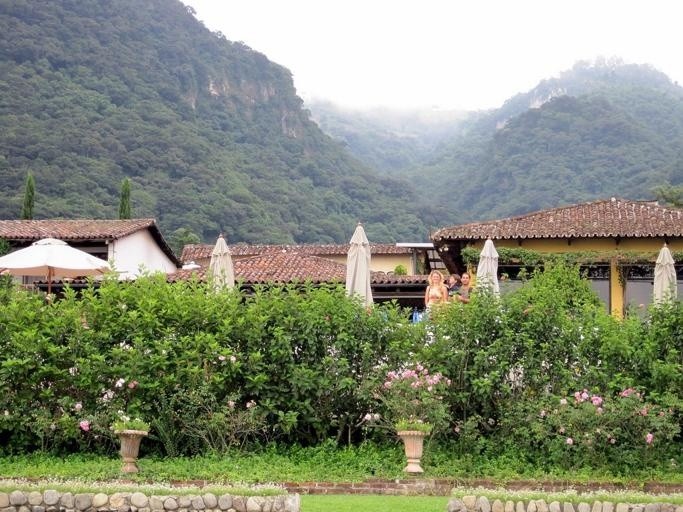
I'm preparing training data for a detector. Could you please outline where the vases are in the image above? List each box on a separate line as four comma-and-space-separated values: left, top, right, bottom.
397, 431, 429, 473
114, 430, 147, 473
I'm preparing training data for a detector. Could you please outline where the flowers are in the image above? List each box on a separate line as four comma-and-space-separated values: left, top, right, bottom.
108, 416, 151, 432
365, 362, 451, 434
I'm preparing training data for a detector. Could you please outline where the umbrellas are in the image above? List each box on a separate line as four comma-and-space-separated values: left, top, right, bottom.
652, 246, 677, 311
205, 231, 235, 302
345, 218, 375, 310
0, 237, 111, 305
472, 239, 500, 301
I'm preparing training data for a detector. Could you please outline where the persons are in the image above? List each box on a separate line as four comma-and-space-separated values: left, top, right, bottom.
423, 269, 448, 321
454, 272, 474, 305
445, 273, 461, 296
